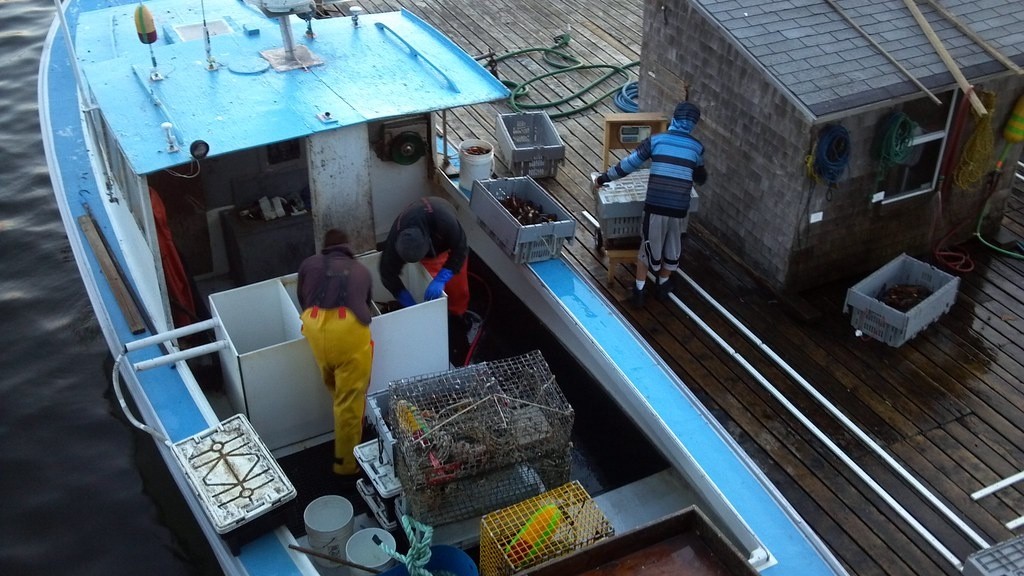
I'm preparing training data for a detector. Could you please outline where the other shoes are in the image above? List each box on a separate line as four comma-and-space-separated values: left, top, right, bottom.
352, 466, 367, 475
633, 280, 648, 296
655, 278, 671, 290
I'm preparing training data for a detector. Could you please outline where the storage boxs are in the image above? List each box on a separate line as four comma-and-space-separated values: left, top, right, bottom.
172, 413, 298, 557
843, 252, 961, 347
495, 109, 566, 179
590, 167, 698, 238
352, 440, 548, 550
469, 175, 576, 265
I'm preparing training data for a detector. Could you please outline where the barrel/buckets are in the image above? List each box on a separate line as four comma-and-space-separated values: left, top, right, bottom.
459, 139, 495, 192
303, 494, 397, 576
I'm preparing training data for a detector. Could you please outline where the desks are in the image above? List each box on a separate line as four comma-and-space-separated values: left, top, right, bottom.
220, 205, 316, 286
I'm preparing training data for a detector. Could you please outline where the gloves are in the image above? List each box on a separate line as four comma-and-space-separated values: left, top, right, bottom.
400, 292, 416, 307
424, 268, 454, 302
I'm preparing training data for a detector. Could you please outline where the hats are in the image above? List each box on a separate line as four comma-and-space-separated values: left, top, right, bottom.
396, 228, 424, 263
324, 229, 348, 247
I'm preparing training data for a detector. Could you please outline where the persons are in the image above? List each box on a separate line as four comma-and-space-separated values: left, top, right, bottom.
296, 229, 374, 494
594, 102, 707, 304
379, 197, 469, 353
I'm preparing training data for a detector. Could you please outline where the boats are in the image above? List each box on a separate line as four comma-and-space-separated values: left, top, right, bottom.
34, 1, 854, 576
